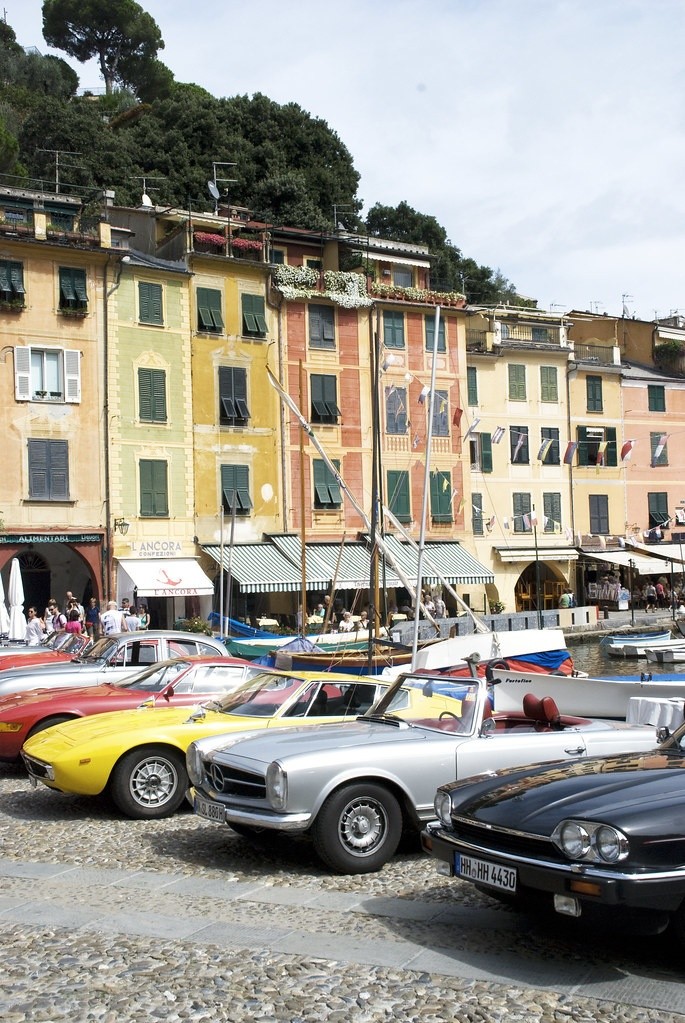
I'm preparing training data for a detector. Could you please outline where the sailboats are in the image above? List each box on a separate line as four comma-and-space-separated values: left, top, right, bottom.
206, 305, 591, 710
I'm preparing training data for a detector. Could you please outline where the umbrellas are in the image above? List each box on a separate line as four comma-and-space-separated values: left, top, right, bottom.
0, 558, 27, 641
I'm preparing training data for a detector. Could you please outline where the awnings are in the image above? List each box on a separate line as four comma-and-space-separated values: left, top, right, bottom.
583, 545, 685, 575
116, 559, 215, 596
501, 555, 578, 562
202, 536, 495, 593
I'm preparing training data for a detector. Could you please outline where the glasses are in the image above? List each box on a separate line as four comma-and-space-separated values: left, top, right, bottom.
140, 607, 144, 609
29, 611, 33, 614
49, 609, 53, 612
122, 602, 126, 604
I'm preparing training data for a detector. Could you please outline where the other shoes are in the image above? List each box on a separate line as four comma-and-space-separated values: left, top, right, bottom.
652, 611, 655, 613
645, 609, 648, 613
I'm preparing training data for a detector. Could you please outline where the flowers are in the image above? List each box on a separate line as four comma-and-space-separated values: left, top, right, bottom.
274, 264, 374, 309
369, 280, 467, 302
193, 231, 263, 253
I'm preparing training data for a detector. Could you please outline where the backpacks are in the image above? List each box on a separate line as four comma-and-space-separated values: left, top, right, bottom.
656, 588, 659, 592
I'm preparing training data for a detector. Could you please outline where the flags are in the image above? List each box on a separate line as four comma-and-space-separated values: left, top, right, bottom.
379, 345, 447, 447
433, 409, 685, 548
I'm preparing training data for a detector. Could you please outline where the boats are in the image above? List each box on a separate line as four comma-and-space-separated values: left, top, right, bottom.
599, 629, 671, 658
675, 611, 685, 637
490, 663, 685, 722
606, 638, 685, 665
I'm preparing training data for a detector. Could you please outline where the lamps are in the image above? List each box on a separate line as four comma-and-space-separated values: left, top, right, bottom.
625, 523, 640, 536
114, 518, 129, 535
473, 518, 492, 533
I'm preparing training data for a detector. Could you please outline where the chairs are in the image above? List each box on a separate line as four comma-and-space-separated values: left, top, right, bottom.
461, 691, 492, 720
509, 694, 561, 733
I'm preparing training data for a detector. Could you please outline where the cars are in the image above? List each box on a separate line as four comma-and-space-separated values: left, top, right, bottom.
0, 634, 188, 671
0, 653, 344, 765
185, 672, 675, 874
19, 671, 499, 821
421, 718, 685, 972
1, 629, 245, 698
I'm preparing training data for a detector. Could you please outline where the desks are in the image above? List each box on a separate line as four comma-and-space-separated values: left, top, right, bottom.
585, 596, 643, 610
515, 581, 567, 611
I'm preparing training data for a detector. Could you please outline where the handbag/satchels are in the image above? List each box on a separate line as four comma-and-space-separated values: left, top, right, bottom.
569, 601, 574, 608
86, 621, 93, 628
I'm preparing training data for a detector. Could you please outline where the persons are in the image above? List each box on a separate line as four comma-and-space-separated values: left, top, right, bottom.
26, 591, 150, 646
339, 601, 370, 632
645, 576, 685, 612
419, 594, 447, 620
389, 603, 416, 619
558, 587, 575, 608
297, 595, 337, 627
